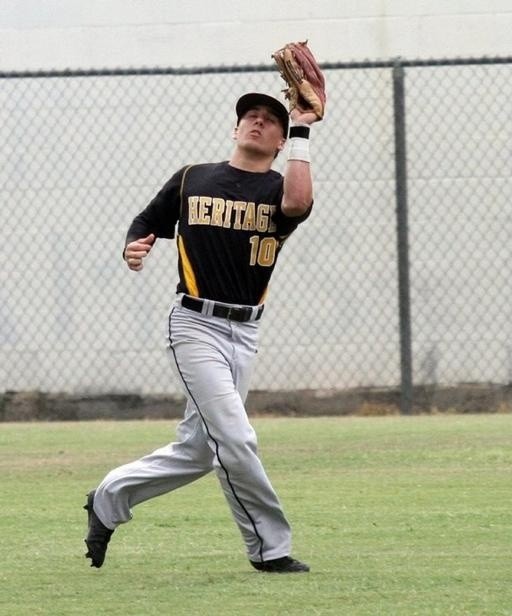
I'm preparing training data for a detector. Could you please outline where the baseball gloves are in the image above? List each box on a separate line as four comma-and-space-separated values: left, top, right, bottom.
273, 41, 326, 123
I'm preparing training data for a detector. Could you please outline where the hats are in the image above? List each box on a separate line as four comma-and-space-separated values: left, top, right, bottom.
235, 93, 288, 139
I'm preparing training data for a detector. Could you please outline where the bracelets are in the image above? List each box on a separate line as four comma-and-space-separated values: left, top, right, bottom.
287, 124, 311, 163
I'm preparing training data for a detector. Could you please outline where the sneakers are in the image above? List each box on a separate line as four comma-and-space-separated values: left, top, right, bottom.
84, 490, 114, 568
248, 555, 310, 572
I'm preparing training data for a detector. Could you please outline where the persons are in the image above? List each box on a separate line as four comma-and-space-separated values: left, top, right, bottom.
83, 92, 314, 573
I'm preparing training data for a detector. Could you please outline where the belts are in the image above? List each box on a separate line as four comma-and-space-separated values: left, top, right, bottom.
181, 294, 264, 323
